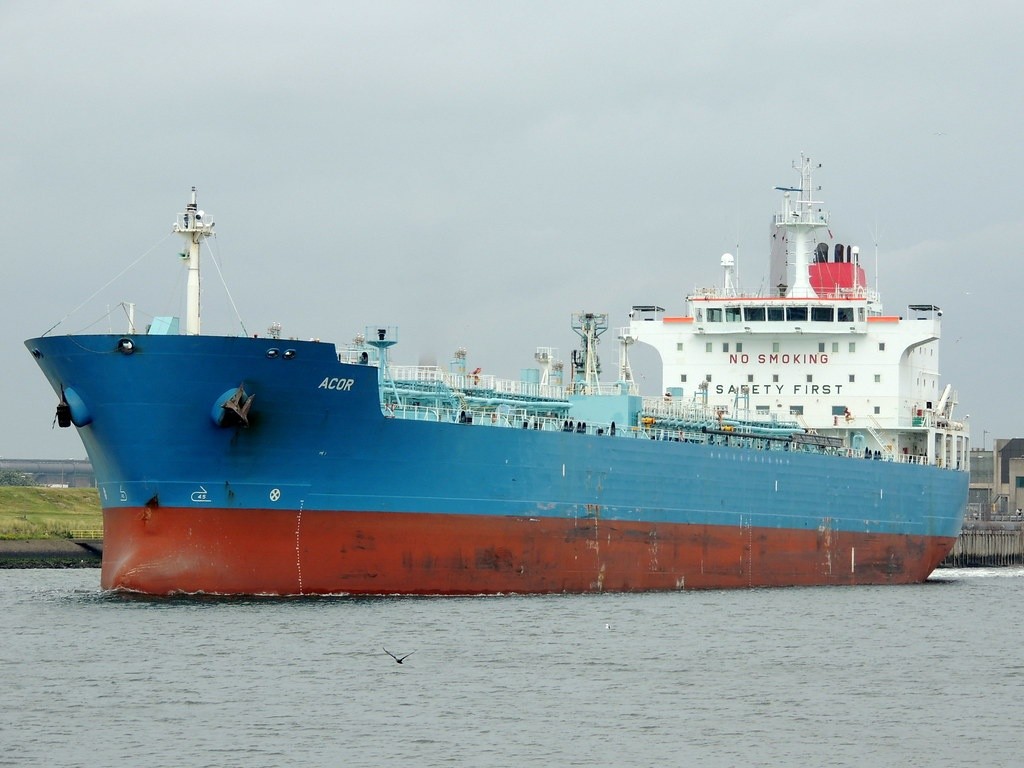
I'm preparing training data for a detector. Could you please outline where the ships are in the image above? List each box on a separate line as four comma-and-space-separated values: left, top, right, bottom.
23, 151, 971, 600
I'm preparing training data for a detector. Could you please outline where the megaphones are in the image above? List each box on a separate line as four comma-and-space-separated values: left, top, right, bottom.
179, 252, 187, 259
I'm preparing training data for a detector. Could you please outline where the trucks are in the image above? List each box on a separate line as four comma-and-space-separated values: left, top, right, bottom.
965, 502, 984, 520
